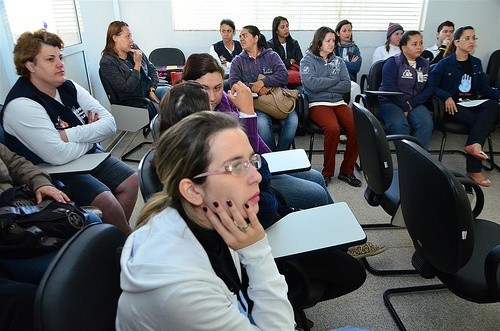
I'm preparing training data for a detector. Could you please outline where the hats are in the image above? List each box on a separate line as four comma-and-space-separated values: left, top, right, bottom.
386, 22, 403, 39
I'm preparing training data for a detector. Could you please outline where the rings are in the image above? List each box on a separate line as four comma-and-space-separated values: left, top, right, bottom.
241, 224, 248, 233
59, 191, 62, 193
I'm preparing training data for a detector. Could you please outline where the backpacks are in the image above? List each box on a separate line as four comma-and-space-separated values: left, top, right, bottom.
0, 183, 89, 261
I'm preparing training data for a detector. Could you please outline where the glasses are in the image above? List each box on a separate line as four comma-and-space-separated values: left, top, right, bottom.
455, 37, 478, 41
190, 153, 262, 178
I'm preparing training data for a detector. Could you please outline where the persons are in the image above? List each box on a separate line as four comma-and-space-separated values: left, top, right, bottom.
302, 26, 362, 188
379, 30, 434, 152
163, 80, 366, 331
0, 28, 139, 233
185, 54, 387, 258
210, 20, 243, 66
0, 143, 102, 283
100, 21, 172, 118
336, 20, 363, 82
116, 111, 296, 331
422, 21, 454, 71
428, 26, 500, 186
262, 16, 304, 69
229, 25, 299, 151
373, 22, 404, 63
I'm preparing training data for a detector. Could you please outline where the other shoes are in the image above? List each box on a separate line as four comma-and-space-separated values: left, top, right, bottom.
465, 144, 489, 160
338, 172, 362, 188
347, 242, 387, 259
466, 174, 491, 187
323, 176, 331, 187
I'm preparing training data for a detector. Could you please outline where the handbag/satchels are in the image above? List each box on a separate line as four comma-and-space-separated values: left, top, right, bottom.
251, 74, 300, 120
287, 63, 303, 86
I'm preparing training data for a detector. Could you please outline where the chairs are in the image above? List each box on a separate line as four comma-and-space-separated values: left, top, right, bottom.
33, 48, 500, 331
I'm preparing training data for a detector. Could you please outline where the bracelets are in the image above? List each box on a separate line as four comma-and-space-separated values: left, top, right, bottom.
149, 89, 155, 92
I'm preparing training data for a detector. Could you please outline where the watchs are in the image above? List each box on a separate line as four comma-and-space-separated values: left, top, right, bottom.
290, 61, 293, 65
